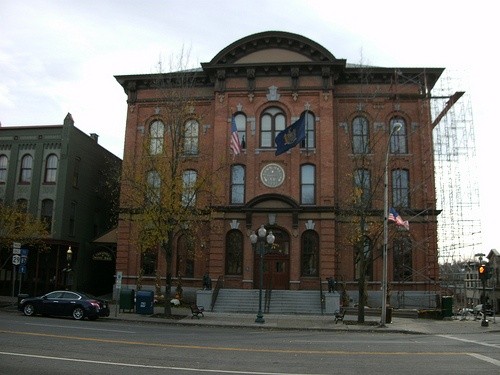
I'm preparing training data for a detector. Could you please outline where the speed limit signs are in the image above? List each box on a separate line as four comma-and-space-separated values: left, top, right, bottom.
12, 255, 20, 265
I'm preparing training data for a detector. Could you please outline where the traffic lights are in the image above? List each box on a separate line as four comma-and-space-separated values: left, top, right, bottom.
478, 262, 492, 280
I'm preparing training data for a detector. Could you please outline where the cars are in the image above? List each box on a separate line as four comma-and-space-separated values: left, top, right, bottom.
18, 290, 110, 321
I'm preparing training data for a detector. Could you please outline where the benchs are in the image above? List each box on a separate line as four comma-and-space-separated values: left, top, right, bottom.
334, 309, 346, 324
190, 304, 204, 318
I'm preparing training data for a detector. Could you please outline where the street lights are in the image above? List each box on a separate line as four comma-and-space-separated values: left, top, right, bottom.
380, 124, 400, 327
250, 224, 276, 322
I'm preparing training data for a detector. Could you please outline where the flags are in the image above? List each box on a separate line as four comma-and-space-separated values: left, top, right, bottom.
388, 207, 409, 230
230, 116, 241, 160
274, 115, 305, 156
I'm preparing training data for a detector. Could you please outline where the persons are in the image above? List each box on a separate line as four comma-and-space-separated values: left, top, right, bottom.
202, 272, 209, 288
326, 277, 335, 293
